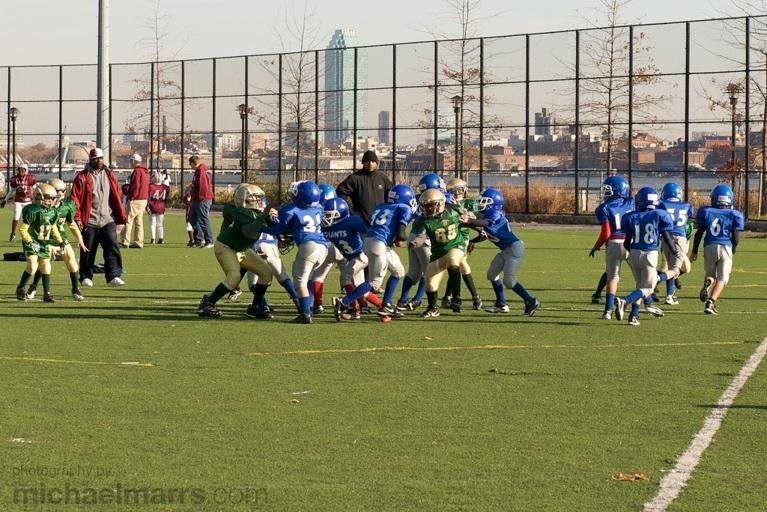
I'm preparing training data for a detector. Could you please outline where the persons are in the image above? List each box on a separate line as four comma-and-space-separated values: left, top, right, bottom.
182, 193, 204, 247
0, 162, 39, 244
188, 154, 215, 249
335, 150, 395, 294
689, 182, 745, 316
196, 171, 541, 323
16, 144, 174, 303
586, 175, 698, 326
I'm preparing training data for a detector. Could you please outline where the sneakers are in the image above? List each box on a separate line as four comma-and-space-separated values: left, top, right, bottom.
16, 277, 83, 302
118, 243, 139, 248
151, 239, 156, 243
107, 277, 123, 285
591, 275, 718, 325
83, 278, 93, 286
158, 239, 163, 243
9, 233, 15, 242
187, 241, 214, 249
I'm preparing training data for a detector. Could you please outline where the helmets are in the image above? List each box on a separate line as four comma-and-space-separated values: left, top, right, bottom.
599, 177, 734, 211
33, 178, 66, 207
234, 174, 503, 227
151, 172, 162, 185
17, 164, 28, 176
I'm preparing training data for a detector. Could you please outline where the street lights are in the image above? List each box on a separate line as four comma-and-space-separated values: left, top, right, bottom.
721, 86, 741, 192
449, 95, 461, 180
8, 107, 20, 177
237, 104, 247, 184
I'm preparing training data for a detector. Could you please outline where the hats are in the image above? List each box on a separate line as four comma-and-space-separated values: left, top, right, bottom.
130, 154, 142, 161
90, 148, 103, 159
362, 151, 377, 162
